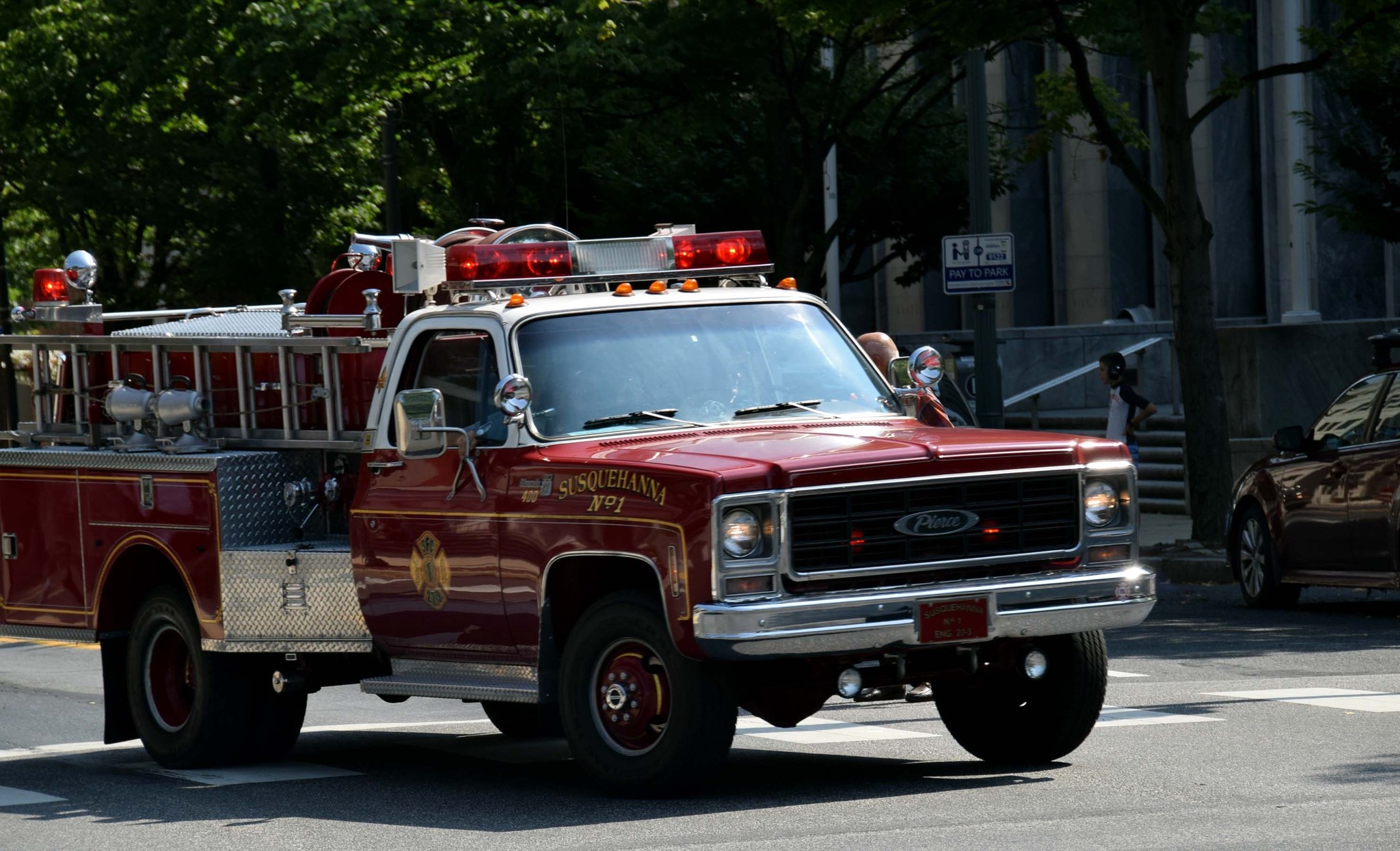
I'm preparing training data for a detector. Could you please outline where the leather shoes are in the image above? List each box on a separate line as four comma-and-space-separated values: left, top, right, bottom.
906, 683, 935, 703
854, 684, 907, 703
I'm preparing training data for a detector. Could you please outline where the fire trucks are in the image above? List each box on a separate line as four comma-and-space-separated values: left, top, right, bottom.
0, 220, 1155, 807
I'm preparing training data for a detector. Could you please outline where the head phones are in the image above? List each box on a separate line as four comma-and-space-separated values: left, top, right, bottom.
1105, 352, 1122, 381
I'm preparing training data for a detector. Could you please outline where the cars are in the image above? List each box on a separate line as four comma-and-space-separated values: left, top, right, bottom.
1223, 370, 1400, 610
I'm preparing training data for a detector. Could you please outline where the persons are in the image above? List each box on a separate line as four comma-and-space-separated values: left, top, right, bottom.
1100, 352, 1158, 472
849, 332, 954, 702
455, 382, 550, 460
